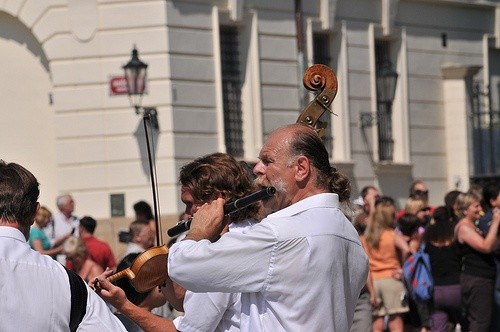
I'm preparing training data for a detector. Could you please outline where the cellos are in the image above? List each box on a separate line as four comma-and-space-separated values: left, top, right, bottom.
297, 64, 338, 138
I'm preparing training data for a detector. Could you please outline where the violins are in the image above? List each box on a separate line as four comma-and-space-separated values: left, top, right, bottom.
89, 244, 171, 292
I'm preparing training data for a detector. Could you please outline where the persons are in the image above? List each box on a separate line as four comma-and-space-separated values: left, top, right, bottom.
113, 252, 166, 332
353, 182, 500, 332
158, 273, 187, 313
166, 124, 369, 332
30, 193, 157, 288
0, 161, 128, 332
88, 153, 260, 332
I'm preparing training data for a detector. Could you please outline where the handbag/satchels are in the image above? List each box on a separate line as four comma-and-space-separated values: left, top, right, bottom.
403, 240, 434, 302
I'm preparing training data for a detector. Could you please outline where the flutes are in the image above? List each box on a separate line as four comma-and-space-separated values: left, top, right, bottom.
167, 185, 276, 237
